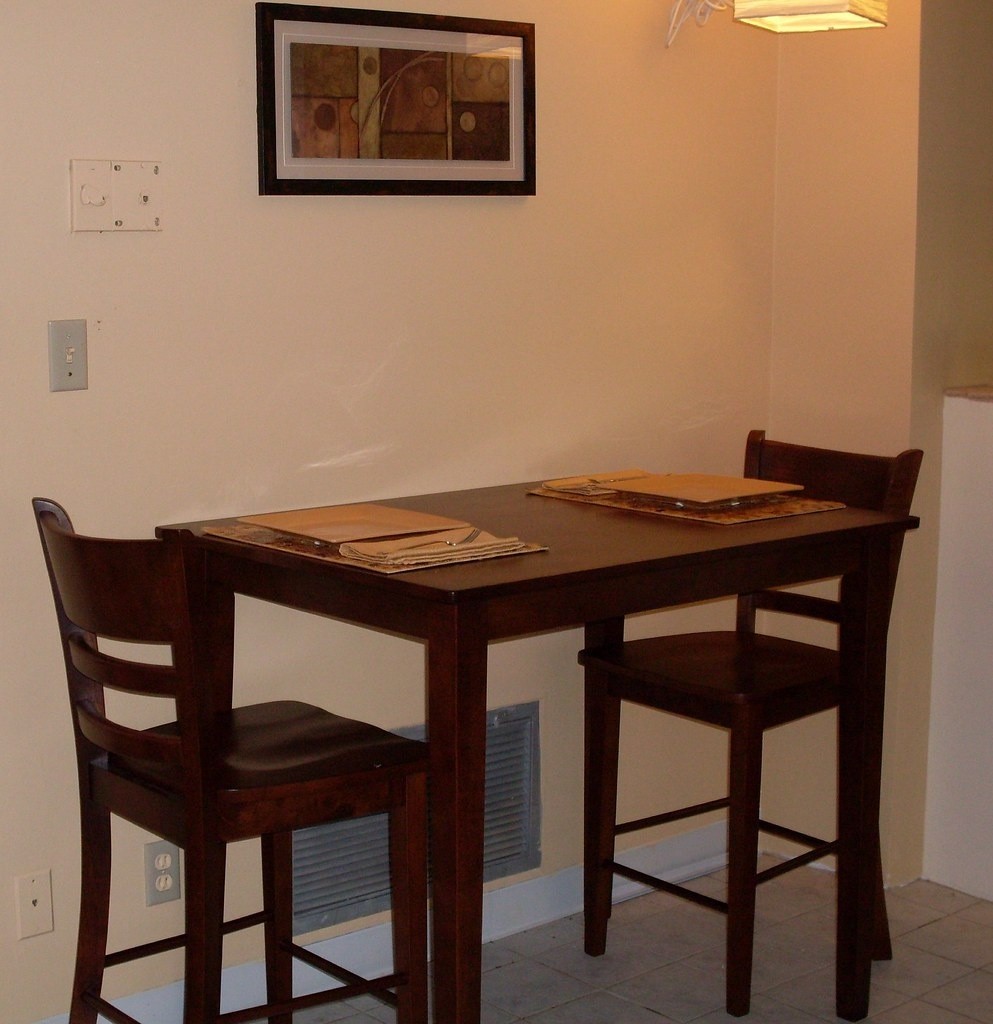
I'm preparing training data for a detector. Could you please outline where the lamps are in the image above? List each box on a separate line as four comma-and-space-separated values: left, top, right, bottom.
733, 0, 888, 34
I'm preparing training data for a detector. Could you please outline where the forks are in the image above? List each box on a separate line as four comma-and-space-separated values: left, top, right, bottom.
376, 528, 483, 556
588, 473, 673, 484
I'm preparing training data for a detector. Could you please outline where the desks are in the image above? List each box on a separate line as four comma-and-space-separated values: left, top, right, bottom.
151, 468, 889, 1024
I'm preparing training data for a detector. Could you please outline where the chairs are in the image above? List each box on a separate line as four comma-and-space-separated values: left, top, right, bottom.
31, 493, 428, 1024
586, 424, 922, 1018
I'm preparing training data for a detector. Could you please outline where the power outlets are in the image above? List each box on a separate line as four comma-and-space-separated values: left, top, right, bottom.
145, 840, 182, 908
13, 868, 56, 941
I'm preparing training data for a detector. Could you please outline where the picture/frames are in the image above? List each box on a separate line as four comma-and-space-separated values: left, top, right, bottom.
255, 2, 535, 197
